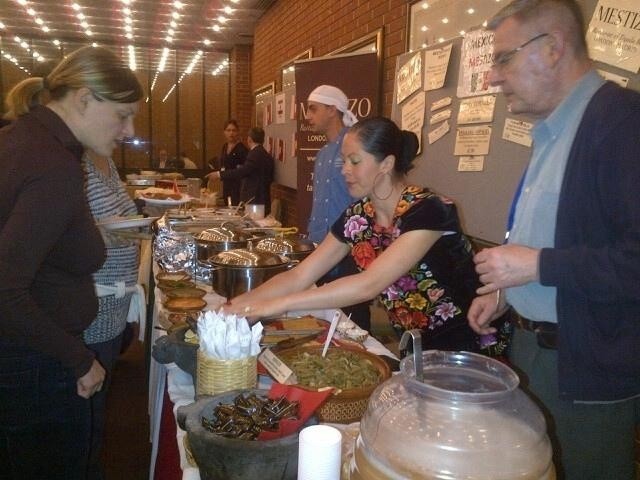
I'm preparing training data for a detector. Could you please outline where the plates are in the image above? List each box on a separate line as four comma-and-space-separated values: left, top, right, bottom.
95, 216, 160, 232
138, 193, 191, 208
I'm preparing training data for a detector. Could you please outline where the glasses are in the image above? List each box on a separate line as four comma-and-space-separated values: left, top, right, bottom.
491, 33, 549, 70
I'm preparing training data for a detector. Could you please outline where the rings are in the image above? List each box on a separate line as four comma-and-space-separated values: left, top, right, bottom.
245, 305, 252, 313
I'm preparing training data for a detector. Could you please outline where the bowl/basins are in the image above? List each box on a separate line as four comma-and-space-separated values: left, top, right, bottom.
336, 321, 369, 343
267, 345, 392, 425
191, 220, 254, 270
156, 271, 208, 334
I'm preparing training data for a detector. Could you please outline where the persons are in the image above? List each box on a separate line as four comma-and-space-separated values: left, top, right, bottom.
181, 153, 198, 170
467, 0, 639, 478
212, 117, 482, 355
152, 150, 175, 169
0, 45, 146, 477
205, 126, 276, 219
303, 83, 372, 337
219, 120, 250, 206
80, 147, 145, 480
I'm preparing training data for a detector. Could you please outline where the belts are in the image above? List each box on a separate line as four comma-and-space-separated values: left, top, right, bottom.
510, 305, 560, 335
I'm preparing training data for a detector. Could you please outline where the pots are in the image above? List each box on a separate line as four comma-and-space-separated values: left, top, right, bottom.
253, 235, 319, 263
206, 241, 301, 301
